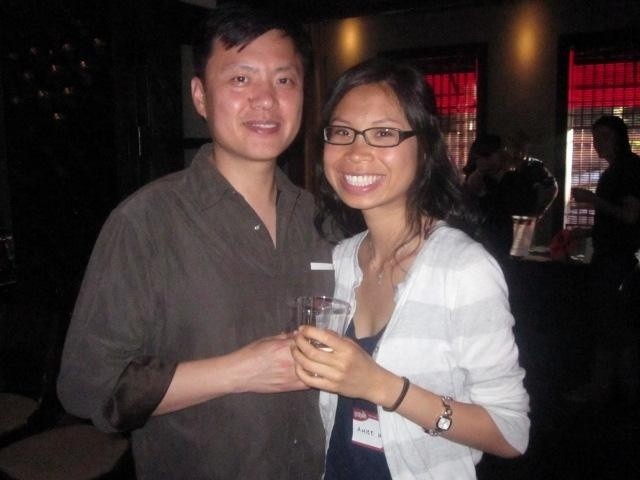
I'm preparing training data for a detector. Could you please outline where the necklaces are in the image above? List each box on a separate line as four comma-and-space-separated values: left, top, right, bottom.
365, 230, 412, 281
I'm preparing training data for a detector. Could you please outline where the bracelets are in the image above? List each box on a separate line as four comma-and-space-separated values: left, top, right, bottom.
382, 376, 409, 413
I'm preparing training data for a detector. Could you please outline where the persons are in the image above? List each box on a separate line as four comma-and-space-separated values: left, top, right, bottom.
560, 114, 640, 406
55, 5, 346, 480
460, 129, 539, 256
288, 57, 533, 479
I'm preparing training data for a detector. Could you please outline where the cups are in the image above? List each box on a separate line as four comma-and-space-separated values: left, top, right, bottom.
296, 295, 350, 352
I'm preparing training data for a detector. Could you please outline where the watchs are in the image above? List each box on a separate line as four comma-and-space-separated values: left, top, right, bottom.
422, 396, 454, 437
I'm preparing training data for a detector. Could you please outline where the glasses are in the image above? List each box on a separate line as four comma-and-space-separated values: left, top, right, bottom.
320, 125, 416, 147
